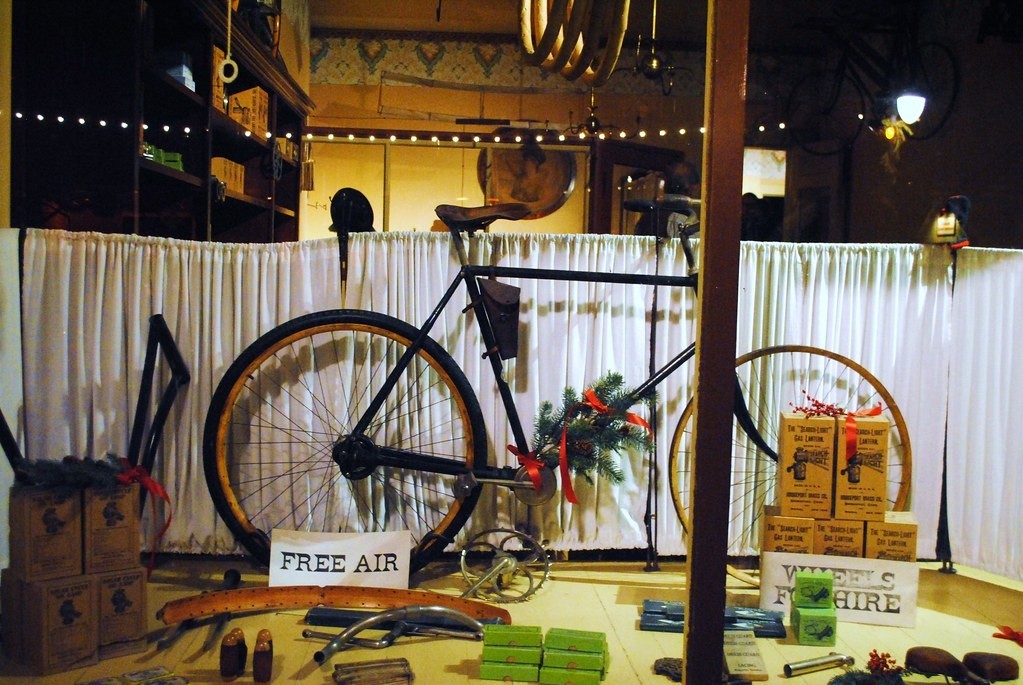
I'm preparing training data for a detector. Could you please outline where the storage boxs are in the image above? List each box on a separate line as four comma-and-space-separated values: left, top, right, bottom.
142, 45, 301, 195
759, 410, 919, 562
1, 482, 149, 678
479, 625, 609, 685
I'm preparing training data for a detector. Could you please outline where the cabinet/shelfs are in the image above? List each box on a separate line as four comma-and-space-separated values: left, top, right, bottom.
10, 0, 317, 244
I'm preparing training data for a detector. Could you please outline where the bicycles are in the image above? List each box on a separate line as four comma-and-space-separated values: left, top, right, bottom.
199, 195, 911, 588
